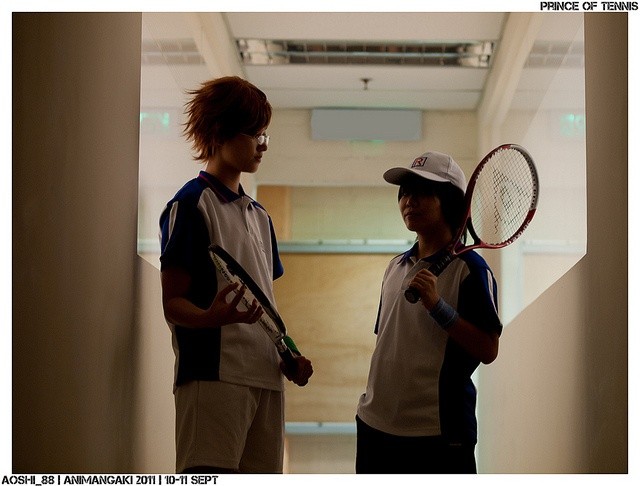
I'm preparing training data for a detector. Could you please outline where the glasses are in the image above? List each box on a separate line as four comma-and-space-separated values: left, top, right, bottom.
241, 130, 270, 145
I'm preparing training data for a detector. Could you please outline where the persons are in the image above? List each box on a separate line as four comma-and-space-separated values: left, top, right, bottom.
158, 76, 313, 474
355, 150, 503, 474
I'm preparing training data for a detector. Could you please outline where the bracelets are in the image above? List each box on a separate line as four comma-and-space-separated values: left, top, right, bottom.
282, 335, 301, 356
429, 298, 459, 330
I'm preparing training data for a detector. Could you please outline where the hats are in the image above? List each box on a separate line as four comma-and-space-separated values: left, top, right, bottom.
382, 150, 467, 196
